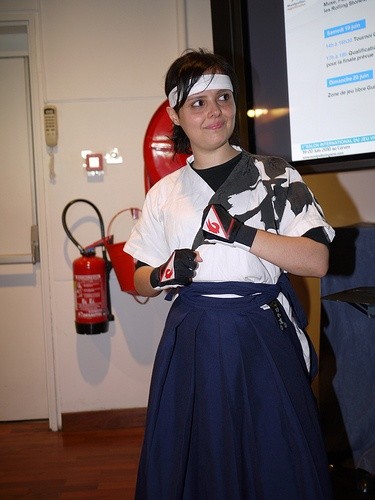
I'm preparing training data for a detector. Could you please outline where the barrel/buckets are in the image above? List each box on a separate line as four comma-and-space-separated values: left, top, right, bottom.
104, 208, 142, 292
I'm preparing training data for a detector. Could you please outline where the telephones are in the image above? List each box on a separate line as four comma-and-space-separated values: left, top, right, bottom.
42, 104, 57, 148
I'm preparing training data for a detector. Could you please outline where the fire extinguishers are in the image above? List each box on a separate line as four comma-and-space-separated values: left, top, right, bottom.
61, 198, 115, 335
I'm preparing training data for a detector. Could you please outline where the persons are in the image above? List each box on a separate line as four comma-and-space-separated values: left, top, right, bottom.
123, 46, 335, 500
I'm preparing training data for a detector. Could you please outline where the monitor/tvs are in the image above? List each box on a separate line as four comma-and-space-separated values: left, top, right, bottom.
229, 0, 375, 173
